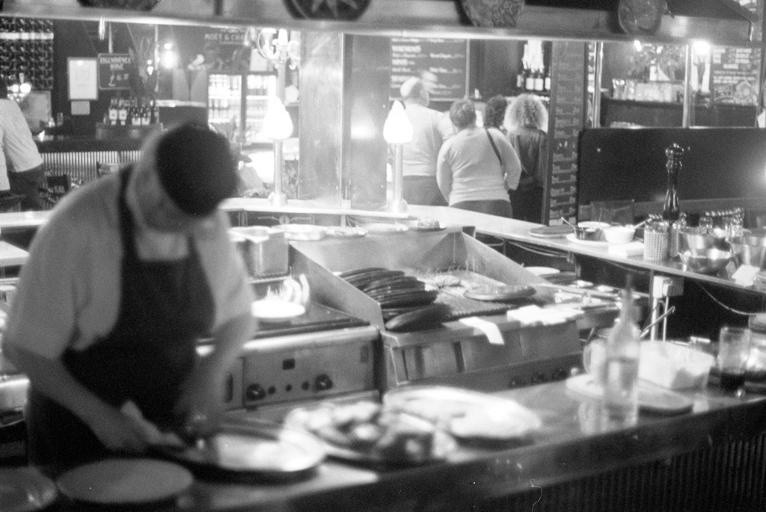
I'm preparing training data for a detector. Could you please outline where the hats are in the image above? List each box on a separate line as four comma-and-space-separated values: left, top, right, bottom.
400, 76, 419, 98
153, 118, 241, 218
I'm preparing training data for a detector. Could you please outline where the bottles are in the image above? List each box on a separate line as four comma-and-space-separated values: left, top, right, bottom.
602, 272, 643, 420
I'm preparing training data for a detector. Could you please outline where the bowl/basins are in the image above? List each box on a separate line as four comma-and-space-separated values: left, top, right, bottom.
564, 224, 637, 249
724, 231, 766, 273
675, 248, 734, 277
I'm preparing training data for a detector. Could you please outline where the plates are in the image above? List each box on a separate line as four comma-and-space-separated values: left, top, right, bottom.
0, 464, 56, 511
615, 2, 668, 38
459, 0, 527, 29
341, 265, 455, 331
301, 402, 455, 465
564, 370, 692, 415
462, 280, 536, 304
526, 224, 574, 239
161, 416, 324, 480
247, 299, 306, 324
288, 0, 373, 22
54, 460, 194, 503
385, 386, 541, 442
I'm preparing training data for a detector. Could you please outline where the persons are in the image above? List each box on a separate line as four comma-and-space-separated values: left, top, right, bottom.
1, 75, 45, 212
482, 93, 510, 128
1, 120, 258, 466
435, 98, 523, 218
503, 91, 550, 224
391, 76, 456, 206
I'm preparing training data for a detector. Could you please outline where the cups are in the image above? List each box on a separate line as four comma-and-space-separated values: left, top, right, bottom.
641, 231, 670, 264
716, 325, 753, 391
